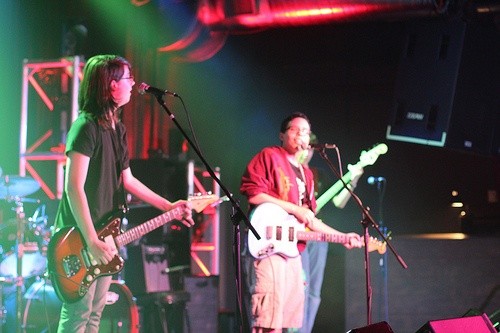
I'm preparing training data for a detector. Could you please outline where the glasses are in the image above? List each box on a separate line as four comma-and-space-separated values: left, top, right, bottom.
122, 74, 136, 81
287, 125, 312, 134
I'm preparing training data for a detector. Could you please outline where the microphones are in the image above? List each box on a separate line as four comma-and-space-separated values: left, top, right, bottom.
137, 82, 176, 98
300, 142, 335, 149
386, 16, 468, 146
367, 176, 384, 185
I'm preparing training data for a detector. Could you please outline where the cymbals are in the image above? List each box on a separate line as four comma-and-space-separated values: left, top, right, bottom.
0, 174, 41, 200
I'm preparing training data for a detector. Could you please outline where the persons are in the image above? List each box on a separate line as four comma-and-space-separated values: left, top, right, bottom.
55, 55, 195, 333
240, 113, 365, 333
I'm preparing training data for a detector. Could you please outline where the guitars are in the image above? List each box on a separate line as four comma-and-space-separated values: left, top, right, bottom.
242, 202, 388, 260
47, 191, 219, 304
310, 143, 388, 216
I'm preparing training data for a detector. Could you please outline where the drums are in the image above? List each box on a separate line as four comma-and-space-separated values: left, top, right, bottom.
0, 281, 20, 333
0, 216, 48, 278
20, 275, 140, 333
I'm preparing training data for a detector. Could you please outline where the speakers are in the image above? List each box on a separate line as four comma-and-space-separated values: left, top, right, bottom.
348, 320, 395, 333
412, 314, 499, 333
171, 273, 219, 332
125, 244, 170, 296
